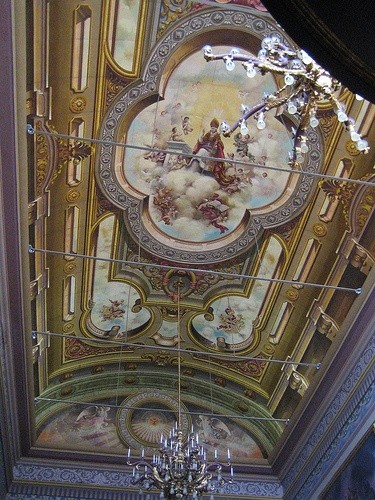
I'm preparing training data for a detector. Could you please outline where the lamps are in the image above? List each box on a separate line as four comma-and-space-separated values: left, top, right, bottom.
203, 34, 374, 173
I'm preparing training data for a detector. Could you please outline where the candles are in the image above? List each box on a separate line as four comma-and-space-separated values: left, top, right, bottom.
127, 423, 235, 498
